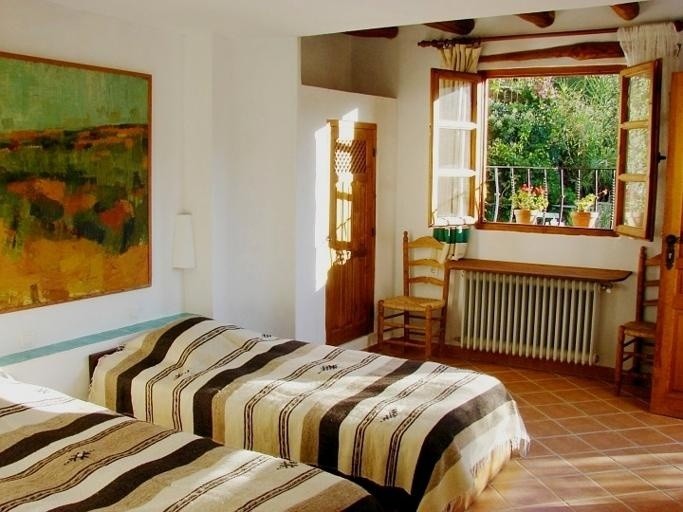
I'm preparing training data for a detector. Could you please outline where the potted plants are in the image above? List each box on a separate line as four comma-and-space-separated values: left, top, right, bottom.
571, 194, 599, 227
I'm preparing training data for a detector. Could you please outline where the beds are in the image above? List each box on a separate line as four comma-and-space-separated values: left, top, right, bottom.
0, 375, 374, 512
86, 314, 531, 511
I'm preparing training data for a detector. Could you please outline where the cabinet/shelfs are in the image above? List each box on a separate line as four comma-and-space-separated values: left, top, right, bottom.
325, 119, 378, 346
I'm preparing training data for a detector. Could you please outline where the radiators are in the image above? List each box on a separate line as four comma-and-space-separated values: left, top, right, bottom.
455, 271, 614, 366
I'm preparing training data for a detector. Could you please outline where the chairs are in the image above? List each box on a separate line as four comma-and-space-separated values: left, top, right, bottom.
378, 231, 450, 359
612, 246, 661, 395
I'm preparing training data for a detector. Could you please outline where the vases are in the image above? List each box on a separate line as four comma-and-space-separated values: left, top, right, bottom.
514, 209, 538, 224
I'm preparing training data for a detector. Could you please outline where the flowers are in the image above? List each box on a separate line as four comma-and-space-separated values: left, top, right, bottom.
509, 184, 549, 211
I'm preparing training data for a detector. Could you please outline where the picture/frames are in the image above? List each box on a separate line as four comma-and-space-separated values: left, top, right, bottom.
9, 53, 153, 314
